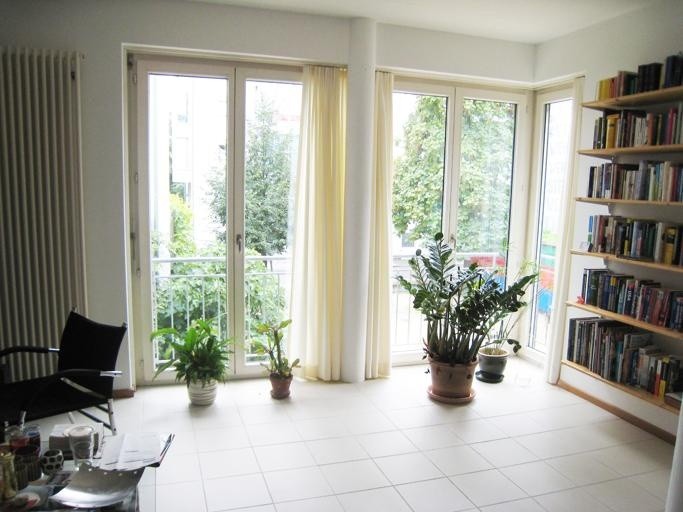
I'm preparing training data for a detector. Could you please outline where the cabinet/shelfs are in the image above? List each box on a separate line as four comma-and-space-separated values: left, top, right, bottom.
562, 85, 683, 418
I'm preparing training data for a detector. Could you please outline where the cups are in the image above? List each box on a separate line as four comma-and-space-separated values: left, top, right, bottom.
12, 444, 40, 491
67, 425, 94, 471
39, 448, 64, 476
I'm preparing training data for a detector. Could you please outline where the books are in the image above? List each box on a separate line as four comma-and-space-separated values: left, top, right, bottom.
593, 103, 682, 149
586, 215, 682, 264
567, 317, 682, 410
581, 268, 682, 332
587, 156, 683, 202
596, 52, 682, 101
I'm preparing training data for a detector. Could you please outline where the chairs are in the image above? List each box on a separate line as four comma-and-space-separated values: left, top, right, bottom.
0, 306, 128, 444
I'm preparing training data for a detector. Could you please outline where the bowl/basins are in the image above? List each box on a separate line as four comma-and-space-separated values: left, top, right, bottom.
47, 462, 145, 509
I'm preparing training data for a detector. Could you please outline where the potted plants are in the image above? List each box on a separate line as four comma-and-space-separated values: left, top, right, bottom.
251, 318, 302, 399
477, 252, 552, 376
394, 232, 542, 405
150, 311, 238, 405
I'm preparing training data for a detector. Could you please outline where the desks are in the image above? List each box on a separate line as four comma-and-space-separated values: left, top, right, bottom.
36, 441, 139, 512
